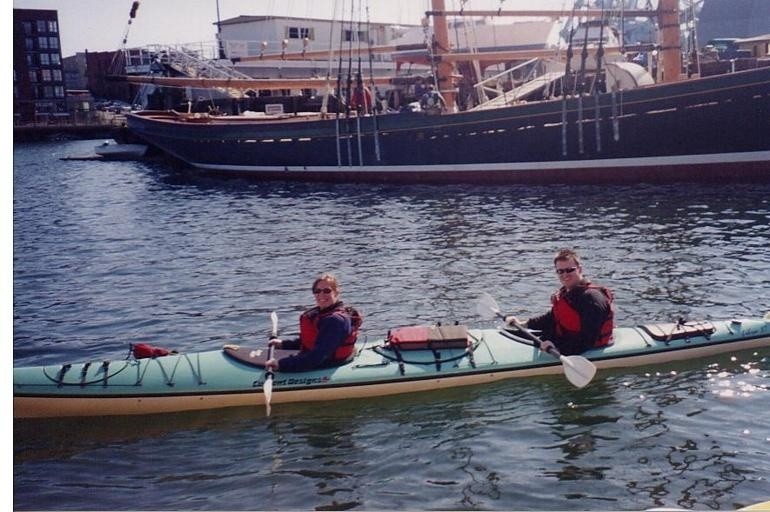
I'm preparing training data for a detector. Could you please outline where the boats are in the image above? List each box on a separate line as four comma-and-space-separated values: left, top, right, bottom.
13, 313, 770, 420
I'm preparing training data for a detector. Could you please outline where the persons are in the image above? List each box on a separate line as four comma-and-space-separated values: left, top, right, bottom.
420, 85, 447, 116
504, 250, 614, 355
265, 274, 363, 373
415, 75, 428, 101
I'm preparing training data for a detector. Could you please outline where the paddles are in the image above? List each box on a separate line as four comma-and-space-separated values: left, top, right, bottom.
263, 311, 278, 416
476, 292, 596, 388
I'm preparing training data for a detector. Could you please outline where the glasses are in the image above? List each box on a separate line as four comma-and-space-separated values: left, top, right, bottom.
557, 267, 577, 274
314, 289, 332, 293
